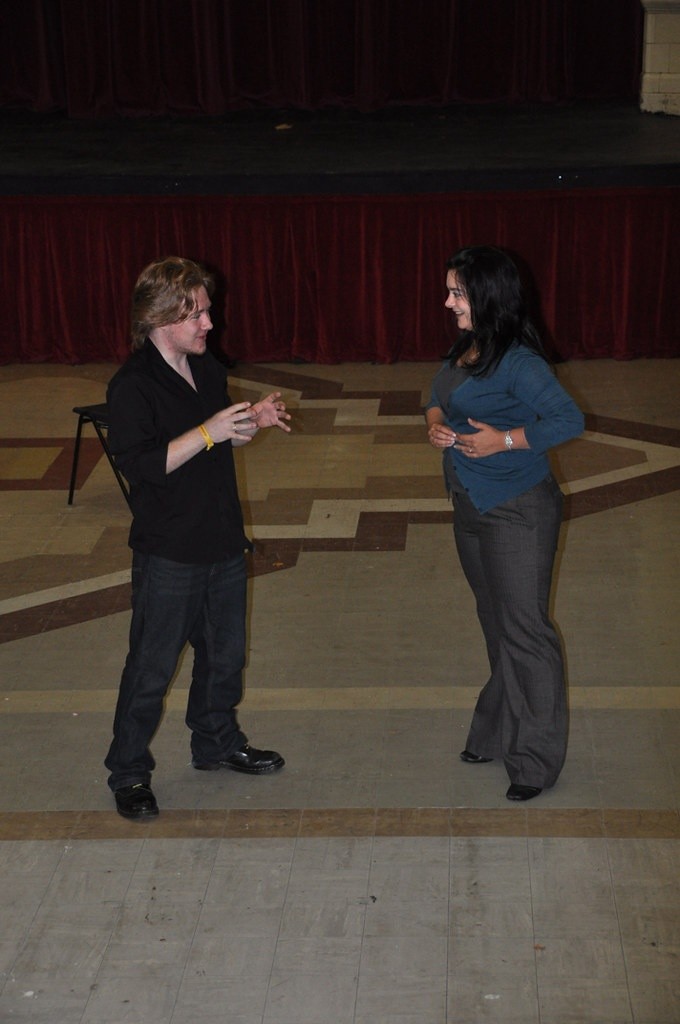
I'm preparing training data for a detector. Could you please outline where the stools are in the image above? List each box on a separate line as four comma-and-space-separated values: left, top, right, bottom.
66, 402, 135, 517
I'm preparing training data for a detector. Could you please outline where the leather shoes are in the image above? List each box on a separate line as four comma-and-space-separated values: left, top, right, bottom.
115, 784, 160, 818
192, 744, 286, 774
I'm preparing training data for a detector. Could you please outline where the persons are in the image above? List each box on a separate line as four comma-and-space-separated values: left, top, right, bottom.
424, 243, 586, 802
104, 257, 292, 818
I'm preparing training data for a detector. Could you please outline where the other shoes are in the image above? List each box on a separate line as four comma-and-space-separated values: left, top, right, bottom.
506, 784, 542, 800
459, 750, 494, 763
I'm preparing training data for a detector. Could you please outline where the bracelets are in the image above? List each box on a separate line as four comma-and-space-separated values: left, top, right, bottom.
198, 423, 214, 451
505, 430, 513, 452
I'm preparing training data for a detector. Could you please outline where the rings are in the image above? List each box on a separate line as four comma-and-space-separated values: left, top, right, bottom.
470, 446, 472, 453
232, 423, 237, 431
